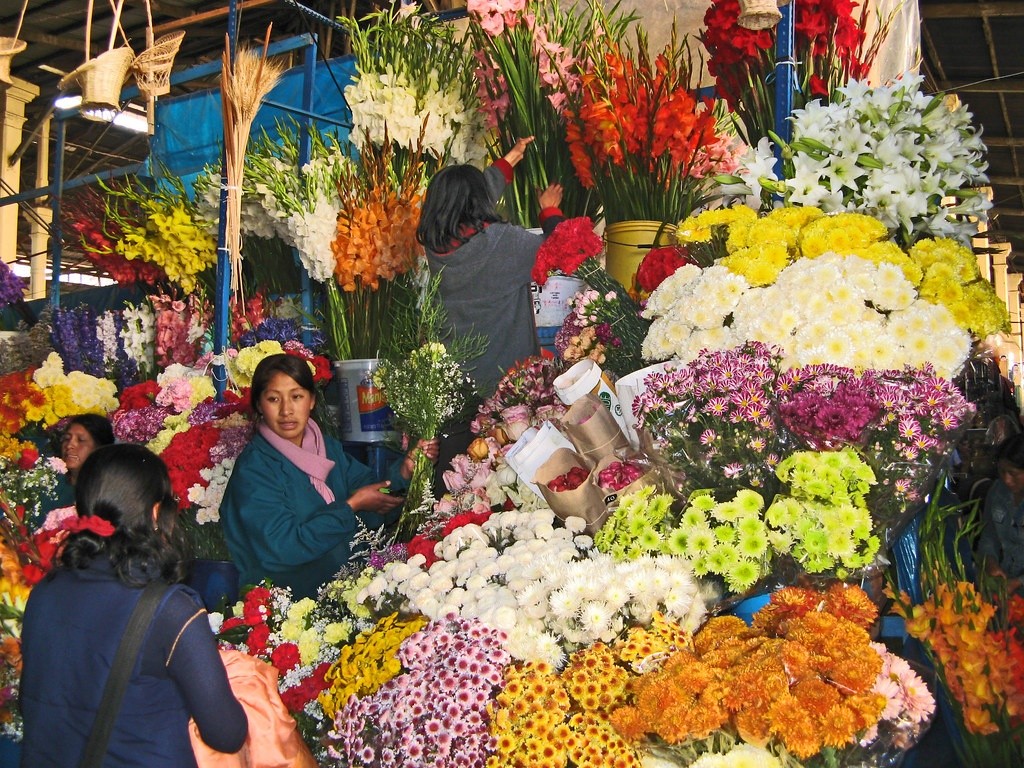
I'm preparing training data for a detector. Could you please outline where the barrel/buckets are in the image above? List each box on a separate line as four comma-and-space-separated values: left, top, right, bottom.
531, 277, 588, 344
602, 223, 679, 294
188, 558, 239, 614
332, 359, 400, 442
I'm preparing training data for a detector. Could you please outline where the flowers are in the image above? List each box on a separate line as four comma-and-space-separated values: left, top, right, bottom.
336, 0, 923, 229
187, 68, 1024, 768
0, 144, 219, 745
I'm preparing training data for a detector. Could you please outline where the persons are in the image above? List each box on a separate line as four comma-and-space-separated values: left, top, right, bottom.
27, 411, 115, 534
219, 353, 442, 601
13, 443, 247, 767
976, 431, 1024, 600
414, 132, 568, 504
